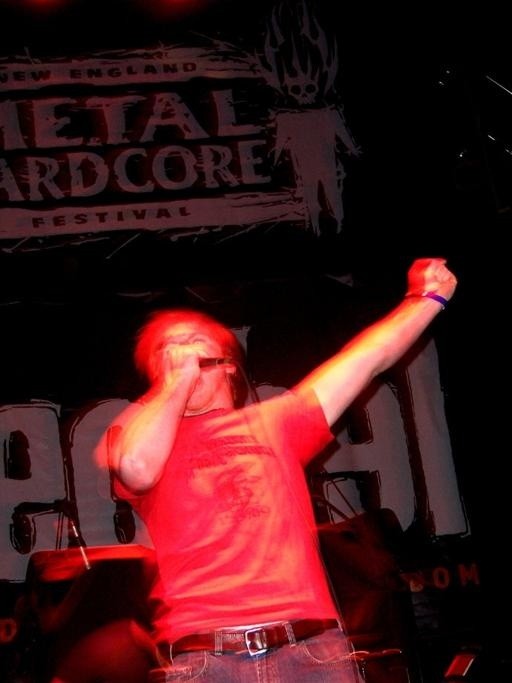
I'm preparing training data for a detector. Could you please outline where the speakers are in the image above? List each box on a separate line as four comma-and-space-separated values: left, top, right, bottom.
399, 540, 511, 680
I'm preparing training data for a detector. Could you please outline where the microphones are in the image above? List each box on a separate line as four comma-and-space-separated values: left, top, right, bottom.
199, 357, 231, 367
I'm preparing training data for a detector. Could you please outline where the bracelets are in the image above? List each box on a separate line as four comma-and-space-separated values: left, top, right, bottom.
420, 291, 449, 311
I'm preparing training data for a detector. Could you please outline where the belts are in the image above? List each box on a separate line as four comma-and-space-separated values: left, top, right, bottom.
156, 618, 337, 658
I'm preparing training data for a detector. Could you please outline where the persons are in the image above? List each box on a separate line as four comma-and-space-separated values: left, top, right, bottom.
108, 257, 458, 683
